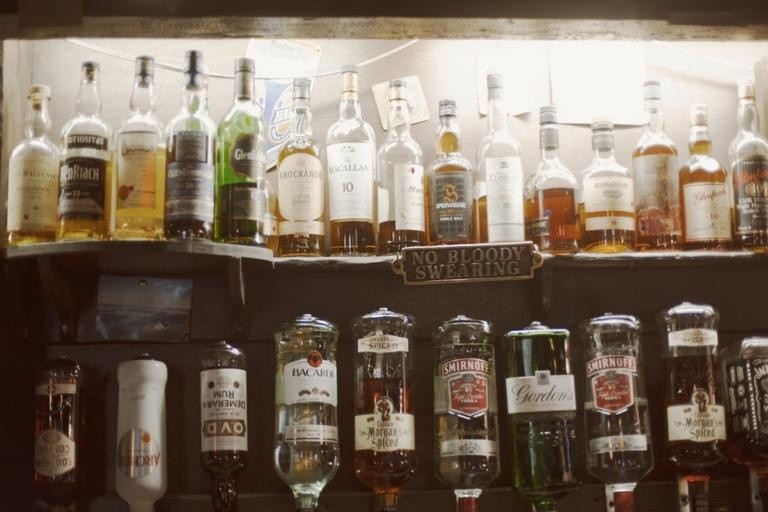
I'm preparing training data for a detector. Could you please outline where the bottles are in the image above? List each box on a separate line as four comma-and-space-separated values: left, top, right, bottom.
271, 313, 341, 511
377, 78, 427, 255
473, 73, 526, 244
214, 57, 268, 247
114, 55, 167, 240
424, 98, 476, 246
526, 105, 581, 254
8, 85, 60, 247
727, 82, 767, 252
114, 352, 169, 511
354, 303, 418, 510
500, 319, 582, 511
580, 312, 655, 512
662, 298, 727, 511
265, 176, 280, 256
631, 80, 688, 252
278, 78, 327, 257
721, 334, 767, 511
328, 68, 378, 257
55, 62, 113, 243
163, 50, 217, 242
677, 102, 737, 252
196, 343, 249, 511
30, 357, 82, 511
432, 316, 501, 510
580, 116, 637, 254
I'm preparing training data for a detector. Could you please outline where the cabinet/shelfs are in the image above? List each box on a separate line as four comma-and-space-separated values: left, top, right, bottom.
0, 0, 768, 512
